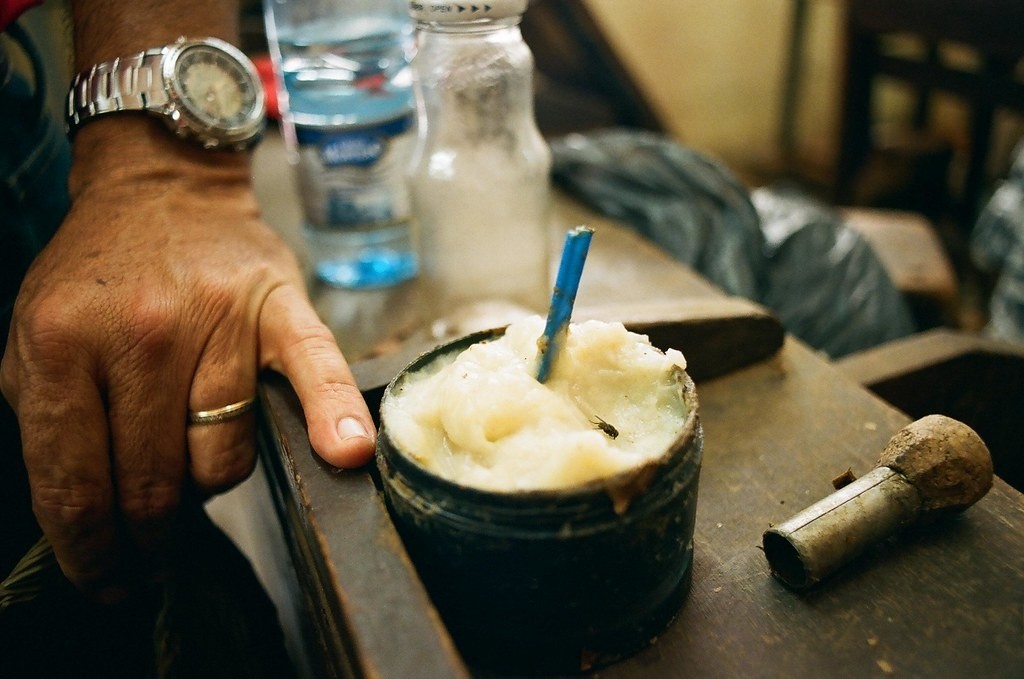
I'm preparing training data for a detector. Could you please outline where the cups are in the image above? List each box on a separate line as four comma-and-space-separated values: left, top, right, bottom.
377, 328, 703, 679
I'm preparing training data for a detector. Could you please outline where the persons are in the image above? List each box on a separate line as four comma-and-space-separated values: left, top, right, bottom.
0, 0, 374, 679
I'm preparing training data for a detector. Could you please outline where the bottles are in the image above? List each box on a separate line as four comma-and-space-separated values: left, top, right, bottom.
408, 0, 552, 340
263, 0, 418, 289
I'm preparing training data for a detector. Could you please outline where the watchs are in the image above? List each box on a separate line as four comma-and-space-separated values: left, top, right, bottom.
64, 34, 266, 155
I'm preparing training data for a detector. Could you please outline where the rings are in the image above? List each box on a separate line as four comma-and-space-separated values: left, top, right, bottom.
189, 397, 256, 424
